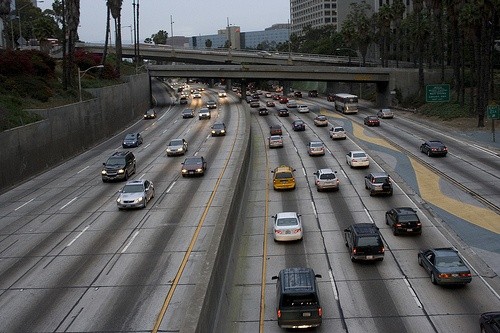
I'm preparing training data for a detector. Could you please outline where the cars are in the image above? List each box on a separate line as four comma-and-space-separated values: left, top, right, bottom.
166, 139, 188, 156
116, 179, 155, 208
122, 133, 143, 148
207, 101, 216, 109
144, 109, 157, 119
182, 109, 194, 119
170, 82, 226, 104
257, 51, 272, 56
181, 156, 207, 177
313, 141, 500, 333
18, 1, 43, 51
272, 212, 303, 242
232, 83, 393, 165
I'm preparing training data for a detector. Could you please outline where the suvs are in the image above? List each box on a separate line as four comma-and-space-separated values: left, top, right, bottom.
198, 108, 211, 120
272, 267, 322, 328
101, 151, 136, 182
271, 165, 296, 190
211, 122, 226, 137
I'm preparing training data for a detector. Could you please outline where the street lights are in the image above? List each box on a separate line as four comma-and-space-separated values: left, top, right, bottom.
78, 65, 104, 101
336, 47, 362, 99
